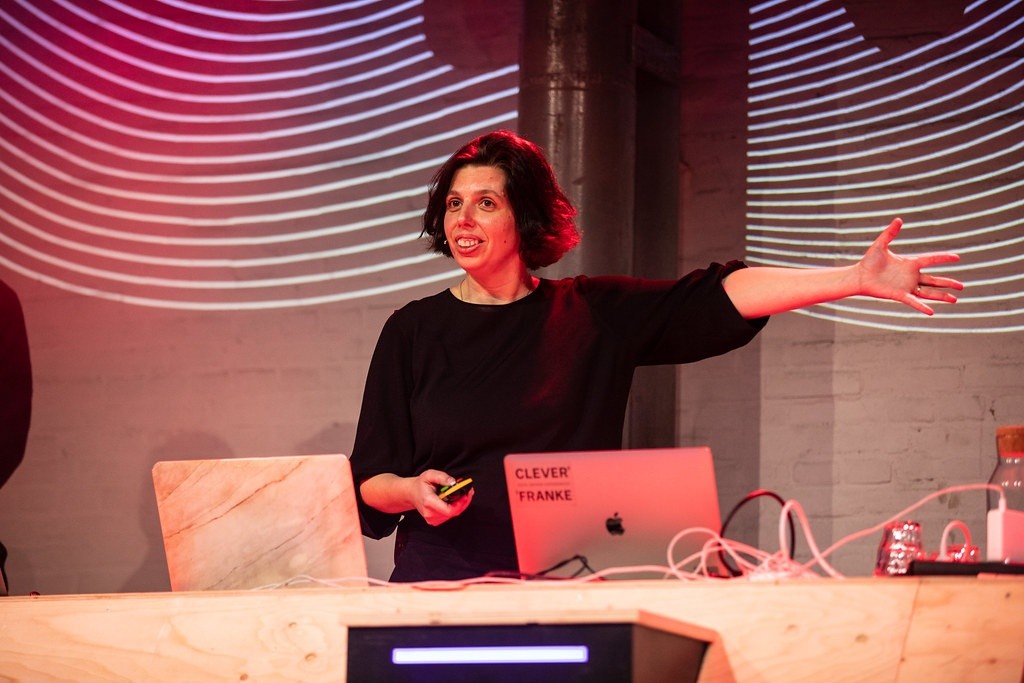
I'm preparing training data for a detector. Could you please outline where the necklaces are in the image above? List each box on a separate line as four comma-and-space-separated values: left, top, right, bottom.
460, 273, 532, 301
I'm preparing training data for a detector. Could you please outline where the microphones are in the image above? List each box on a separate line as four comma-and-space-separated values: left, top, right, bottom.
444, 240, 448, 244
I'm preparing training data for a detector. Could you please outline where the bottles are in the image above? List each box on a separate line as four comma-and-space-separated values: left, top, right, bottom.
986, 425, 1024, 513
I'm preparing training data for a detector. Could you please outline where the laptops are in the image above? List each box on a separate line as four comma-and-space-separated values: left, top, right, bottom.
504, 446, 723, 580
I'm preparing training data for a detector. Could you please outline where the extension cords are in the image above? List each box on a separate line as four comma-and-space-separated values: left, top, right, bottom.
908, 560, 1024, 576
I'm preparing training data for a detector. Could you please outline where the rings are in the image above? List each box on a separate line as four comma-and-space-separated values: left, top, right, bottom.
916, 285, 921, 295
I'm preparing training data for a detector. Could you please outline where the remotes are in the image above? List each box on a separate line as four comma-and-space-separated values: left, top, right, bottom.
439, 478, 474, 503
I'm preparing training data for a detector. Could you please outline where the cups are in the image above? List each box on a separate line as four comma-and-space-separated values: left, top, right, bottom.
873, 521, 923, 575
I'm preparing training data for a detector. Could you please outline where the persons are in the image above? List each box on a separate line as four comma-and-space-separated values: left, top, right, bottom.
349, 131, 964, 584
